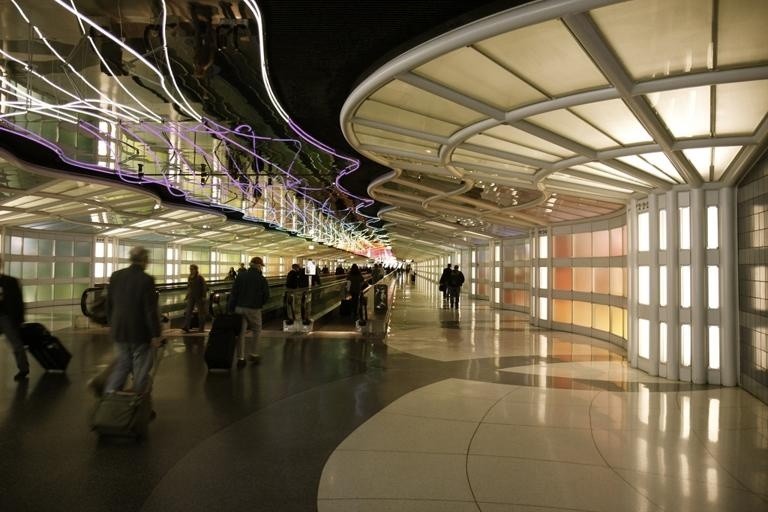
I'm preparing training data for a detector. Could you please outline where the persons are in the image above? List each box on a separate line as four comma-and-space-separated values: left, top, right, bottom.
227, 256, 271, 368
237, 262, 246, 273
285, 263, 300, 290
298, 260, 417, 292
87, 1, 307, 212
345, 263, 365, 317
180, 263, 209, 333
438, 263, 453, 299
0, 273, 31, 381
100, 242, 162, 423
445, 264, 465, 310
227, 267, 237, 280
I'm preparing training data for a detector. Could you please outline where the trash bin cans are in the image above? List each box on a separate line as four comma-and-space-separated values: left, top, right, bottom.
73, 314, 88, 328
371, 318, 385, 335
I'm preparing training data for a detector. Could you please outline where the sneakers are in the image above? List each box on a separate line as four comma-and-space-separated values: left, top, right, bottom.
237, 355, 263, 367
15, 370, 29, 380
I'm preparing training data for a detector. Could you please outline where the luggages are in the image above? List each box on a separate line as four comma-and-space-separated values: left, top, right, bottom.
19, 322, 72, 370
339, 290, 352, 317
204, 314, 242, 369
89, 340, 168, 437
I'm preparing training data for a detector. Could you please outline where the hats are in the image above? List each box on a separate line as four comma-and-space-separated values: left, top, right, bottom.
251, 257, 265, 266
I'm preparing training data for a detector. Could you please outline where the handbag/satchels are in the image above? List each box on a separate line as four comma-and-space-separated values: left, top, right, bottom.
439, 285, 443, 290
212, 314, 242, 334
